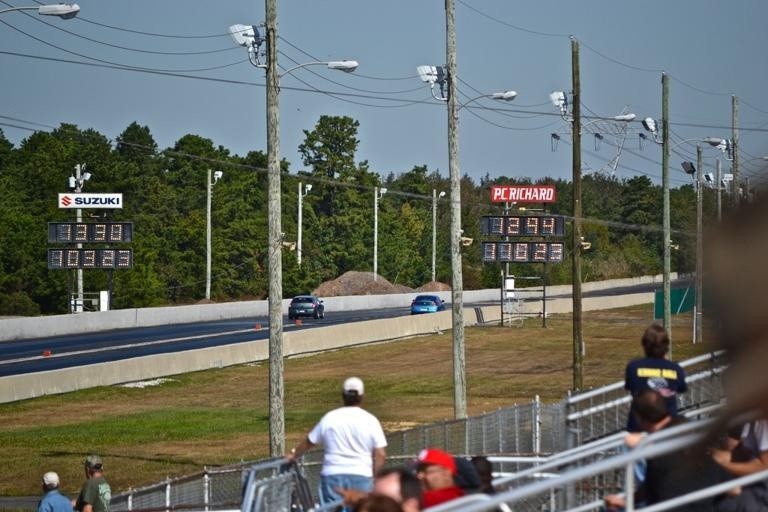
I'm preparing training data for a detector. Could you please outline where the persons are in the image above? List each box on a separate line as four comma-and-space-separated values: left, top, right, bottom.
37, 471, 73, 512
331, 447, 498, 512
70, 453, 112, 512
314, 300, 324, 318
590, 197, 768, 511
285, 375, 390, 512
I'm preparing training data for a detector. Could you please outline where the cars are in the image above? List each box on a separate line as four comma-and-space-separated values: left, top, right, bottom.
410, 295, 445, 315
288, 295, 325, 320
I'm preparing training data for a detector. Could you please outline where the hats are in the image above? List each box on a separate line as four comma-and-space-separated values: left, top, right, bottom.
408, 447, 458, 477
43, 471, 60, 485
343, 377, 365, 396
81, 454, 103, 468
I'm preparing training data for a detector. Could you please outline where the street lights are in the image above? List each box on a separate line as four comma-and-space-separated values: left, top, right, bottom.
415, 0, 517, 420
68, 164, 93, 313
225, 0, 359, 457
373, 186, 388, 282
548, 38, 637, 391
640, 73, 768, 362
205, 168, 223, 300
432, 188, 447, 282
296, 181, 313, 268
